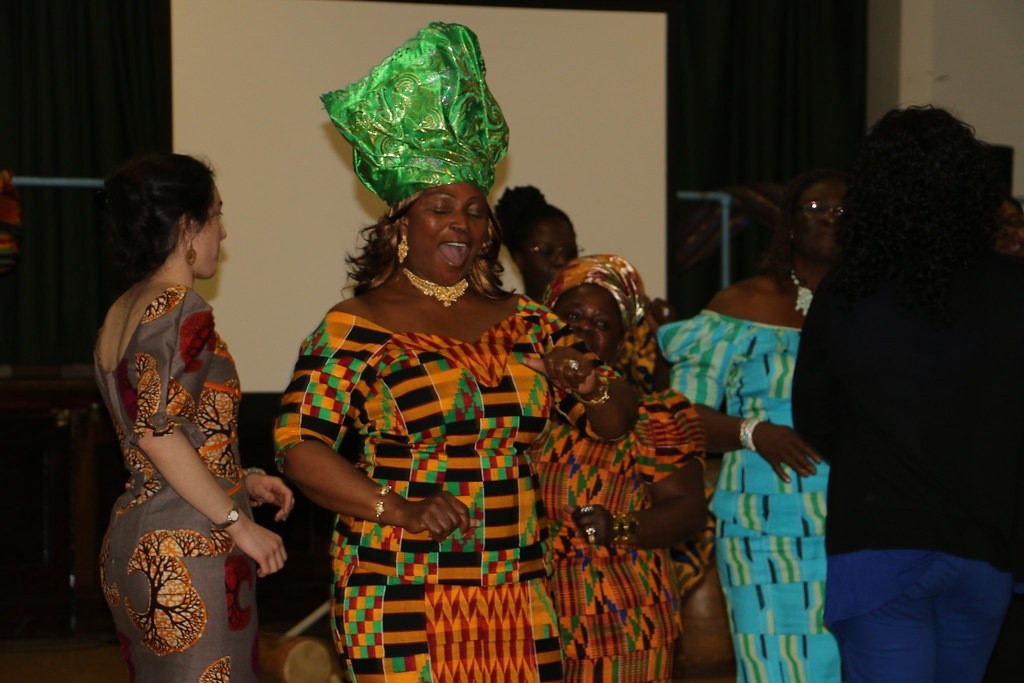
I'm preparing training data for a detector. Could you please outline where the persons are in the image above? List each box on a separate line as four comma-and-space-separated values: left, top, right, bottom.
658, 159, 859, 683
90, 152, 297, 683
530, 257, 710, 683
988, 191, 1023, 255
789, 102, 1023, 682
493, 183, 680, 390
268, 156, 639, 683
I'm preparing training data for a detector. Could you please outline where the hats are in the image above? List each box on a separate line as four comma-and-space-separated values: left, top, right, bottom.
534, 250, 660, 390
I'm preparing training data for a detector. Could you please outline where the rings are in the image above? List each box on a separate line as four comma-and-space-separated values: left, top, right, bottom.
662, 306, 670, 316
583, 525, 597, 535
587, 535, 596, 544
579, 504, 594, 514
566, 358, 580, 371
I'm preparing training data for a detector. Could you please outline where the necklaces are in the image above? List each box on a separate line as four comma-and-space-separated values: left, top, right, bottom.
784, 262, 814, 317
399, 266, 470, 306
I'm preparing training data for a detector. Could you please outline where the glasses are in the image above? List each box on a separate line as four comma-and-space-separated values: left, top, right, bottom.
796, 198, 852, 220
520, 242, 585, 260
986, 210, 1024, 230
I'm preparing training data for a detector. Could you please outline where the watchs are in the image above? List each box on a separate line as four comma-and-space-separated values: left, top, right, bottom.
212, 495, 240, 529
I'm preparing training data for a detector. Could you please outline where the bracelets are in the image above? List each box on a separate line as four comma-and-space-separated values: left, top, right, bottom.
374, 483, 393, 524
738, 416, 762, 454
574, 369, 613, 406
612, 510, 639, 545
